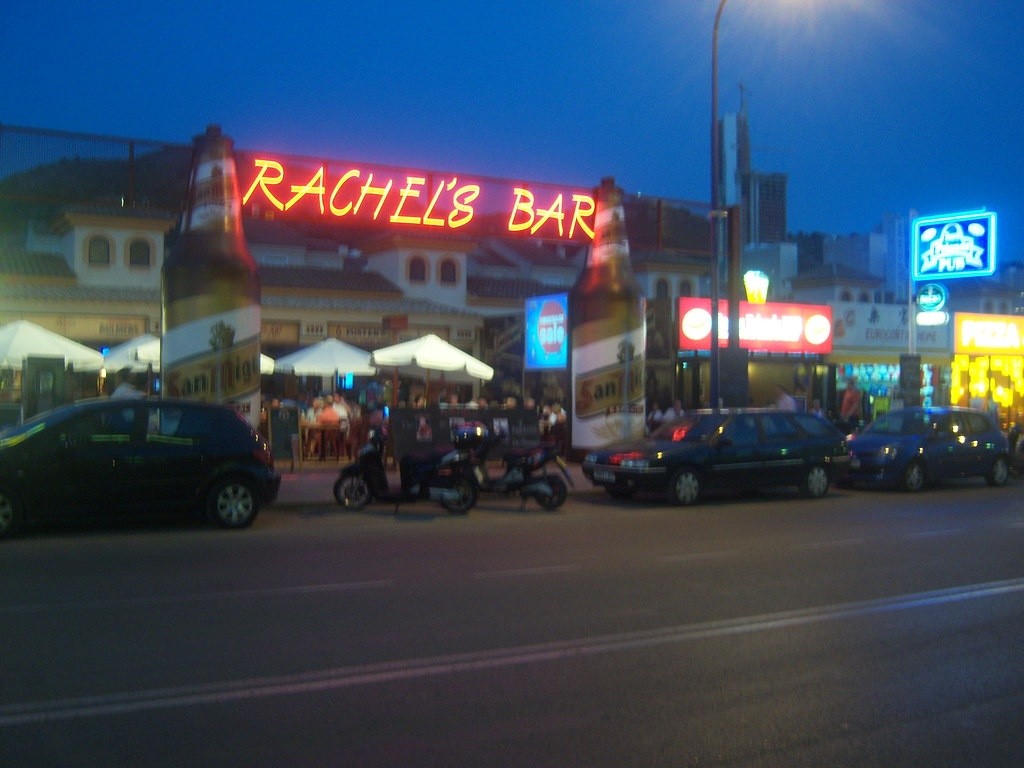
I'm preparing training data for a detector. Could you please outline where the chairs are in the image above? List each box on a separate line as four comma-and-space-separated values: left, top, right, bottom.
301, 421, 362, 463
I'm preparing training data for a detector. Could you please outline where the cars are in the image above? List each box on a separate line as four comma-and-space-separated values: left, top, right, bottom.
0, 394, 283, 541
1009, 423, 1024, 476
836, 403, 1011, 494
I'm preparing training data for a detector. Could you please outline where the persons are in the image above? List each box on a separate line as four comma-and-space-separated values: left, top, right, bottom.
774, 385, 797, 411
842, 380, 860, 428
985, 393, 998, 423
646, 399, 684, 431
308, 393, 389, 459
415, 393, 567, 439
810, 399, 824, 416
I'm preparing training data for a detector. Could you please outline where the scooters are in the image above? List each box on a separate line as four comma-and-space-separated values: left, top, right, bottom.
450, 417, 577, 515
333, 405, 491, 519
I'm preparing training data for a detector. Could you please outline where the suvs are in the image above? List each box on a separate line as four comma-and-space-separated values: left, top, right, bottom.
581, 407, 858, 507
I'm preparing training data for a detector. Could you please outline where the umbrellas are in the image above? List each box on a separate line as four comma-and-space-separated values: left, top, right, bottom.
274, 338, 379, 394
105, 335, 274, 397
371, 334, 494, 406
1, 320, 105, 371
